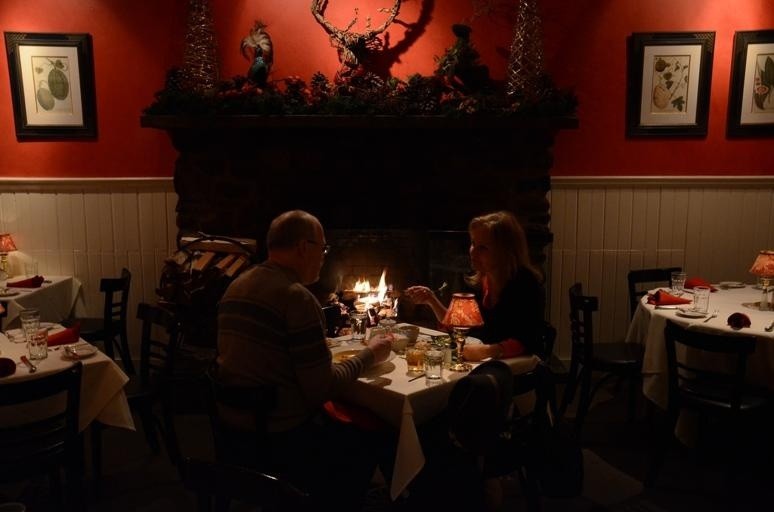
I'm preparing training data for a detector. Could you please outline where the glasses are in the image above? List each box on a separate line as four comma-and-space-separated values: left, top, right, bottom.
306, 240, 330, 255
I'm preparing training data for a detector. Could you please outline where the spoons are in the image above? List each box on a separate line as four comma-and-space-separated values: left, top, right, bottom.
20, 355, 35, 372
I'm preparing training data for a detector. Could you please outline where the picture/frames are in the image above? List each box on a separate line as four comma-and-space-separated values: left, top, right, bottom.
625, 32, 715, 138
4, 31, 98, 140
726, 29, 774, 138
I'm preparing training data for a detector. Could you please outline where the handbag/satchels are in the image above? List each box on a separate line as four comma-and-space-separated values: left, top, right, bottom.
533, 425, 584, 497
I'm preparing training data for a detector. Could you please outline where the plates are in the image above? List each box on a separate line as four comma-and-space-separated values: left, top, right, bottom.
0, 289, 19, 296
334, 348, 398, 368
62, 341, 95, 362
675, 308, 706, 318
721, 280, 746, 289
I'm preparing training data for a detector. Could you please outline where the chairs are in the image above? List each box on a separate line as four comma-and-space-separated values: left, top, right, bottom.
207, 382, 270, 512
514, 321, 557, 430
75, 268, 135, 377
89, 302, 186, 492
0, 362, 83, 512
628, 268, 683, 424
664, 319, 770, 496
558, 281, 645, 427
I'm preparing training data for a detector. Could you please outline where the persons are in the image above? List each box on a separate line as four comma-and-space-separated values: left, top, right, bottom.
404, 211, 546, 512
217, 209, 396, 512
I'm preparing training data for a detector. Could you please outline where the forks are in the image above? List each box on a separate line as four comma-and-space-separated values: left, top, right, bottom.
45, 346, 60, 352
703, 308, 724, 327
403, 281, 449, 295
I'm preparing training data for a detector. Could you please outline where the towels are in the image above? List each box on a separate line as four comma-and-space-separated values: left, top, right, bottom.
684, 277, 720, 292
646, 289, 693, 306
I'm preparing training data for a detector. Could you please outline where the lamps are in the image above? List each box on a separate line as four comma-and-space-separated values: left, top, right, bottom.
749, 250, 774, 311
0, 233, 18, 278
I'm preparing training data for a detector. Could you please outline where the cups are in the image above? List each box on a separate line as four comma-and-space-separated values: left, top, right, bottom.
23, 261, 40, 278
19, 308, 50, 358
693, 284, 710, 313
671, 268, 688, 295
405, 344, 426, 374
423, 346, 448, 382
349, 309, 367, 340
399, 325, 418, 345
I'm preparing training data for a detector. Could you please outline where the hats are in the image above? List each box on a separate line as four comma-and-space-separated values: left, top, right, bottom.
449, 360, 514, 434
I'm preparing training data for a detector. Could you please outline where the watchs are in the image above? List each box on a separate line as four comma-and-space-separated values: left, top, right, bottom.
492, 343, 504, 359
